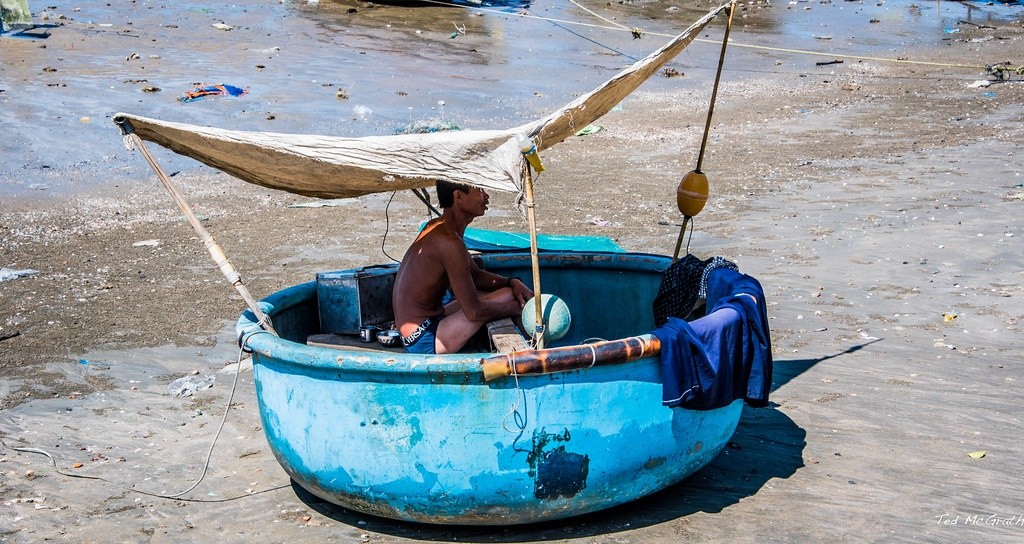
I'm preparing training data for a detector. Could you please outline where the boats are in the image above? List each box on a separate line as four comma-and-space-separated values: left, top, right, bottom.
114, 0, 766, 526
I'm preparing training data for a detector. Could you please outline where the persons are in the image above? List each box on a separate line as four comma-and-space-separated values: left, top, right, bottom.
393, 179, 535, 355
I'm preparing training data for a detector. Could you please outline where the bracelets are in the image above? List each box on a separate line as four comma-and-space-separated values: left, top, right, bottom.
507, 277, 523, 288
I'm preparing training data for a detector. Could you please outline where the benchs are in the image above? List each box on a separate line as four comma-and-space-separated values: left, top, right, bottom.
484, 317, 529, 354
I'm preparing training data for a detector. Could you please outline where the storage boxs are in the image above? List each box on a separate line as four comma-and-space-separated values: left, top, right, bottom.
316, 264, 399, 334
